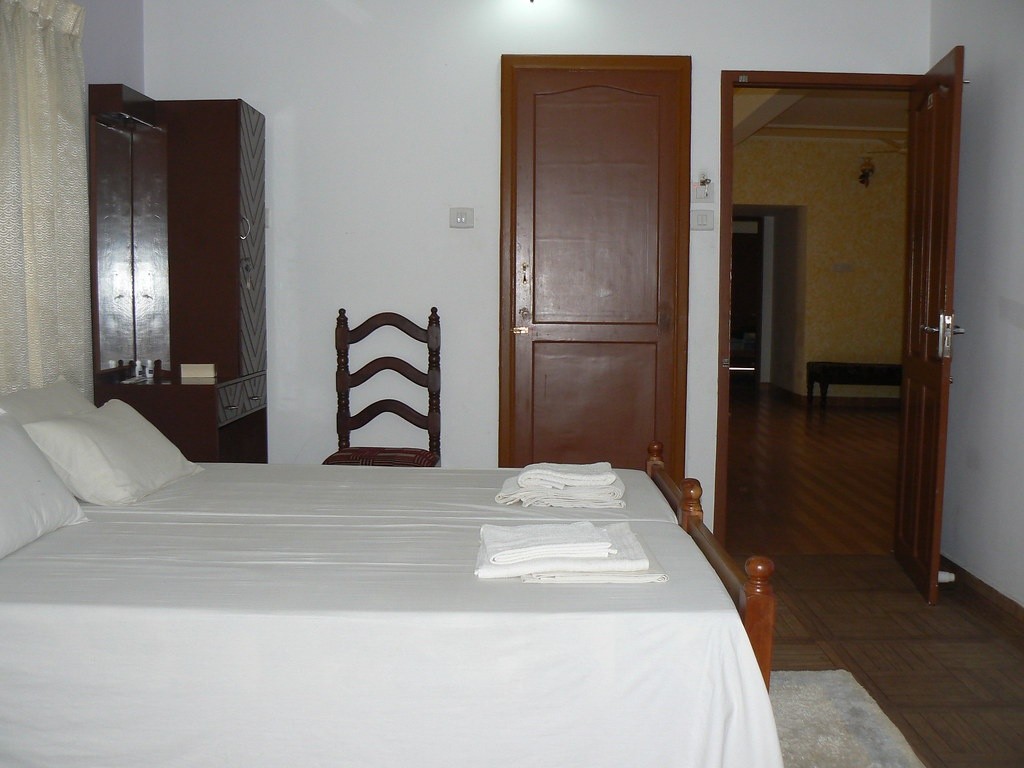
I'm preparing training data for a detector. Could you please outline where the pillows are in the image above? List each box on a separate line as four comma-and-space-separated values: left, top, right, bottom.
0, 408, 91, 560
24, 398, 205, 506
0, 376, 97, 424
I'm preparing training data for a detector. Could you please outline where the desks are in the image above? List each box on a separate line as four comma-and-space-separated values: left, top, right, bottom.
807, 361, 901, 415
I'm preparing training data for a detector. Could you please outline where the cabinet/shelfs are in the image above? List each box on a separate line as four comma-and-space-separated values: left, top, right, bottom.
87, 84, 269, 463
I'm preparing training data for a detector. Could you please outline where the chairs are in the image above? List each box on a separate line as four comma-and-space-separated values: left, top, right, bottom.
322, 307, 441, 467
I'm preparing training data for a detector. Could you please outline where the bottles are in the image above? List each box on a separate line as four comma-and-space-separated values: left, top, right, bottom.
146, 360, 153, 378
136, 361, 143, 377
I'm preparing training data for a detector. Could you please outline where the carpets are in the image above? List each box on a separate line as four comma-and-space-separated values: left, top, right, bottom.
769, 669, 927, 768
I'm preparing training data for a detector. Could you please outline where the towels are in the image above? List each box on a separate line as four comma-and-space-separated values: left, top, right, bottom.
496, 462, 627, 509
473, 522, 668, 585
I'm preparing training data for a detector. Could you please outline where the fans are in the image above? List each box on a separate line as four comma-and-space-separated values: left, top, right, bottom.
867, 136, 909, 155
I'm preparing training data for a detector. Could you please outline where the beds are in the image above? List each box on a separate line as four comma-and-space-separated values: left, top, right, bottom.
0, 442, 787, 768
